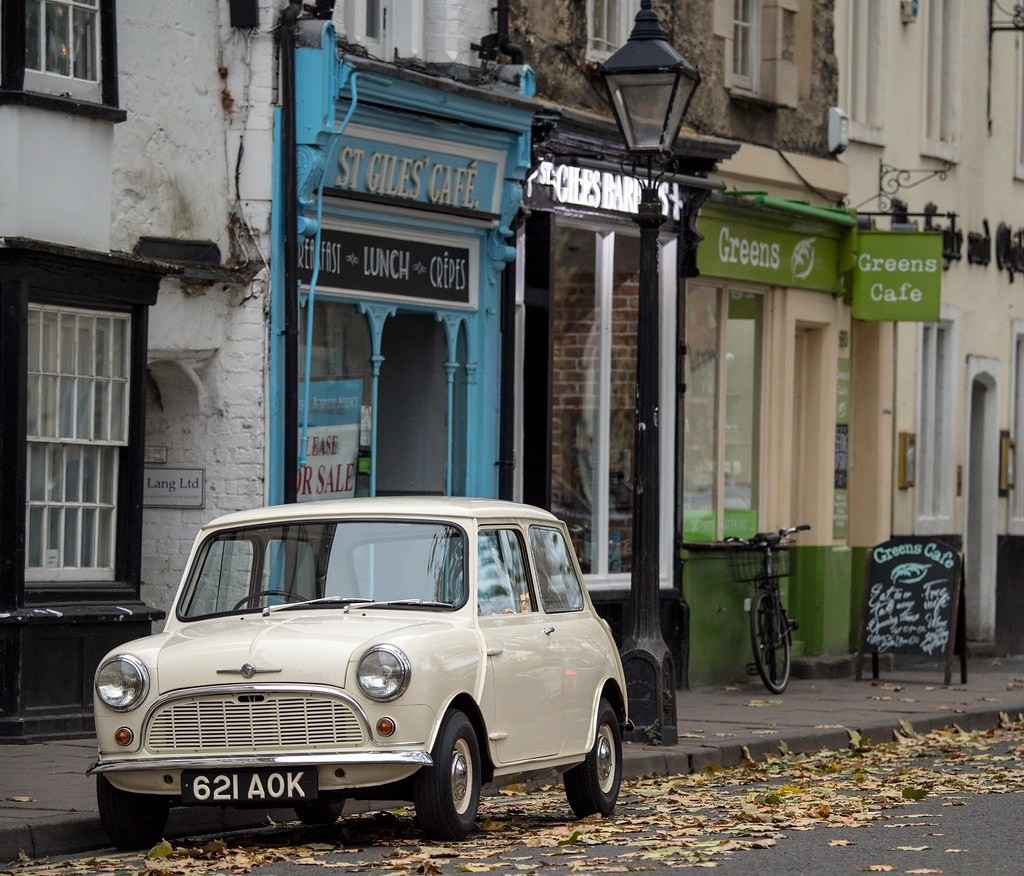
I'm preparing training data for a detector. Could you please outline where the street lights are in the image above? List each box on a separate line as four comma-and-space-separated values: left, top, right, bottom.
593, 1, 701, 745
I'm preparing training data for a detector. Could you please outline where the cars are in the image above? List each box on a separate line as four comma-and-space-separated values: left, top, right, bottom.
86, 496, 636, 848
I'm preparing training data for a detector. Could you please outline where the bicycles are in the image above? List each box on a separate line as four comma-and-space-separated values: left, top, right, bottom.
723, 524, 812, 695
